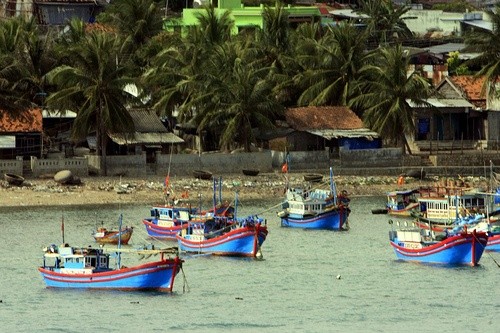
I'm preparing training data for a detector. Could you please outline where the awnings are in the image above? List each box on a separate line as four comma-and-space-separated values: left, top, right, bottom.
0, 105, 77, 135
107, 130, 186, 147
283, 104, 382, 141
404, 98, 470, 113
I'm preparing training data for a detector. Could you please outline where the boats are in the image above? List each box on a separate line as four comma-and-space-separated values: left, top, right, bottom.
276, 166, 352, 231
175, 189, 268, 258
140, 176, 235, 239
36, 214, 186, 292
90, 211, 133, 246
387, 219, 488, 268
386, 185, 500, 252
